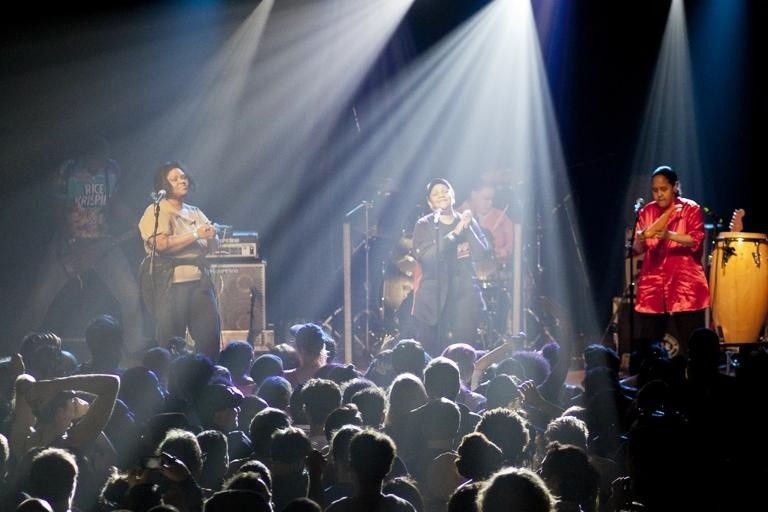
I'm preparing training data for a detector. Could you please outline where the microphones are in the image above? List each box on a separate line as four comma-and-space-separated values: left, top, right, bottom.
156, 189, 167, 205
632, 197, 645, 215
433, 208, 443, 224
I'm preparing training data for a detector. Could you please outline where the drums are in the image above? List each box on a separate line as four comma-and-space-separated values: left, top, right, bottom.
709, 232, 767, 343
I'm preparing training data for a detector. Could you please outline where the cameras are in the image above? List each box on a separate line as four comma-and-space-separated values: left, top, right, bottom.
141, 456, 164, 471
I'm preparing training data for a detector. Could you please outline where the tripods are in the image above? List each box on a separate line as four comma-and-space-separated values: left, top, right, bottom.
320, 230, 557, 363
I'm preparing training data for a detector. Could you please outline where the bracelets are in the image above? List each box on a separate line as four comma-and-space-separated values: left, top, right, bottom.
193, 232, 200, 241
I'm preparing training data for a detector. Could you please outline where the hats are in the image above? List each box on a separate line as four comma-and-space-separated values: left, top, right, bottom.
192, 384, 244, 410
291, 323, 324, 347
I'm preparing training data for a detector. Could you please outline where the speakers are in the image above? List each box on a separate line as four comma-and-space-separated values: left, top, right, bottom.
205, 263, 266, 331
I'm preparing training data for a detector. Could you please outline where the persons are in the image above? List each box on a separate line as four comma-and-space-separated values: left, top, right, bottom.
456, 178, 515, 338
628, 164, 712, 365
136, 161, 223, 361
408, 177, 491, 356
10, 136, 143, 356
0, 315, 768, 512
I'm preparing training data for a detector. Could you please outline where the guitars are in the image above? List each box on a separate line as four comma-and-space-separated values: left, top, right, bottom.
63, 227, 136, 287
